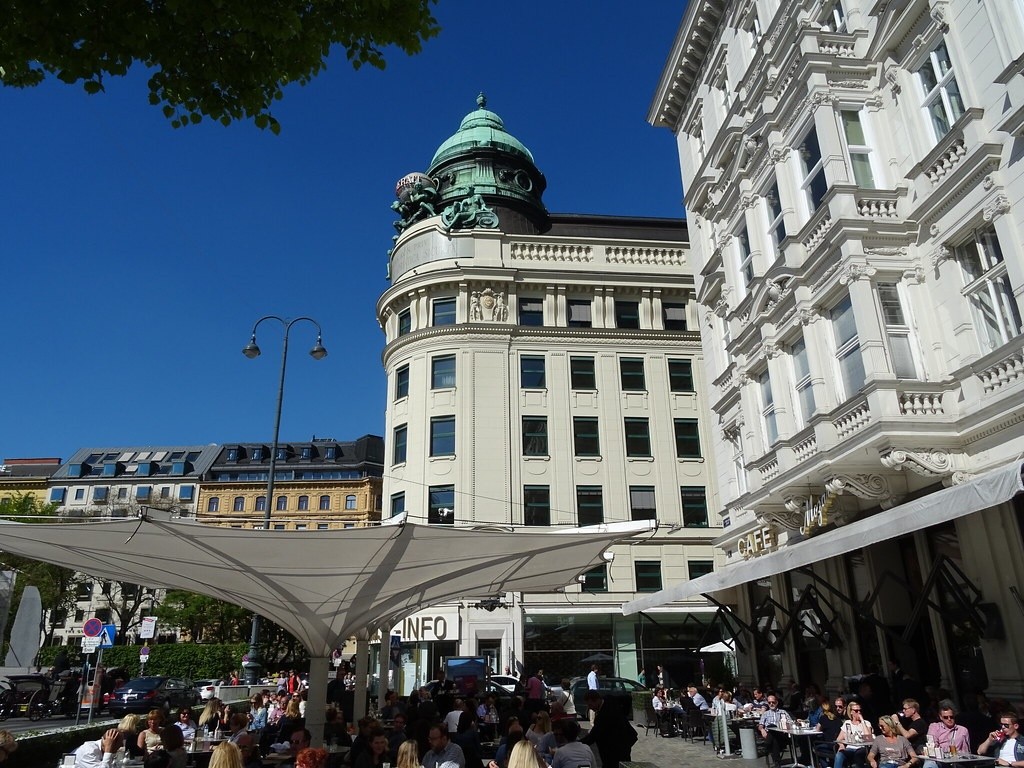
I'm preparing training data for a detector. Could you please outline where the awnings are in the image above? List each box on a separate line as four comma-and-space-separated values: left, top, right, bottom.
620, 458, 1024, 618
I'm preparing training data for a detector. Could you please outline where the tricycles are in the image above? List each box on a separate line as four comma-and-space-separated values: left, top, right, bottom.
0, 669, 81, 723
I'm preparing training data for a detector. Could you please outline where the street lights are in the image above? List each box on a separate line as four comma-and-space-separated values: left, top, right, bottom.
241, 316, 330, 688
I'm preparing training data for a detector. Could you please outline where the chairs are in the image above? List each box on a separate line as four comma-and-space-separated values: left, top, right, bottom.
642, 702, 981, 768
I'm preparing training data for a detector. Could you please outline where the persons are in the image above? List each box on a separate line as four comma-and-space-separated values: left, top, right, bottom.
52, 650, 70, 680
71, 653, 1024, 768
0, 730, 18, 768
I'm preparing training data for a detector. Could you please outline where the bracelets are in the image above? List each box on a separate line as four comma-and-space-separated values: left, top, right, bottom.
907, 761, 913, 766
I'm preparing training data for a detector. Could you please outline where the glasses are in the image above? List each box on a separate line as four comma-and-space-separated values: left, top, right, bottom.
293, 695, 299, 697
238, 744, 251, 751
507, 716, 518, 721
536, 716, 543, 720
533, 744, 539, 749
768, 702, 777, 704
878, 724, 886, 728
289, 740, 304, 745
999, 723, 1014, 728
181, 712, 190, 715
941, 716, 954, 719
428, 737, 441, 742
851, 709, 862, 713
250, 702, 258, 705
903, 707, 912, 711
294, 762, 303, 768
835, 705, 844, 708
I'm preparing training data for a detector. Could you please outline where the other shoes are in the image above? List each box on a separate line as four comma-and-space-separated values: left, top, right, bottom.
758, 747, 769, 755
771, 764, 781, 768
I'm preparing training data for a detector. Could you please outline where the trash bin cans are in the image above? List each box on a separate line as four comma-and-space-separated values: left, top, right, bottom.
739, 724, 758, 759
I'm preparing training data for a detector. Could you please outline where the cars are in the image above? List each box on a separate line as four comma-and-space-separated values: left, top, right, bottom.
548, 676, 651, 722
190, 679, 226, 703
227, 677, 263, 686
421, 675, 524, 722
101, 675, 202, 719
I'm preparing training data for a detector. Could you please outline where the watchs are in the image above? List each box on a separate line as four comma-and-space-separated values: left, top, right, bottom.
1009, 761, 1013, 767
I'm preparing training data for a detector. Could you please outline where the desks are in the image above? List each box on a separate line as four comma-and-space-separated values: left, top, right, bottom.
766, 724, 823, 767
832, 739, 879, 768
913, 751, 996, 768
62, 724, 504, 768
